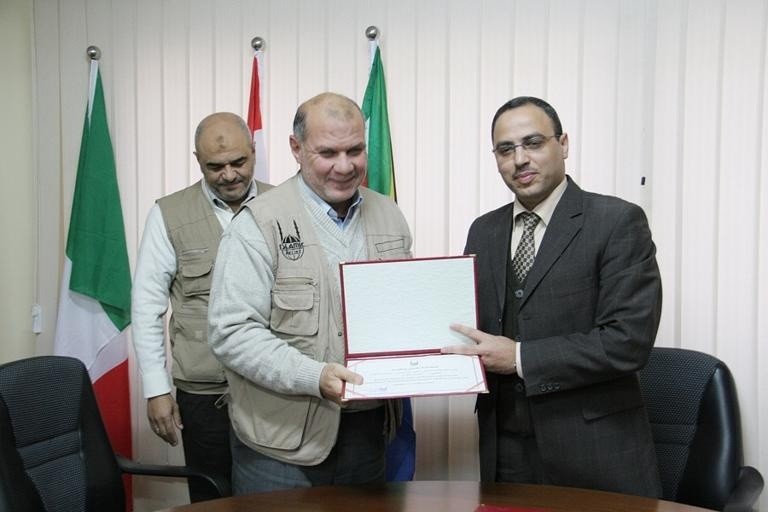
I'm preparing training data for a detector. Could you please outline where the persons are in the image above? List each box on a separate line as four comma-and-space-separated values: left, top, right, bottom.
130, 115, 278, 502
206, 92, 413, 498
441, 97, 664, 502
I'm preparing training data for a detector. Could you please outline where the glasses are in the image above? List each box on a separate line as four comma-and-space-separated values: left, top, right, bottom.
490, 134, 564, 162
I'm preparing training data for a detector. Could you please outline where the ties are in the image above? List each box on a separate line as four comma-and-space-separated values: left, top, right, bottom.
510, 213, 543, 286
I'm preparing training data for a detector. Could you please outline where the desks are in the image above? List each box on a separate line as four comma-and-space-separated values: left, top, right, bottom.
159, 478, 723, 510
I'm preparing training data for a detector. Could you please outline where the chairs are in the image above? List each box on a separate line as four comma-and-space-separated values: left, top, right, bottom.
637, 344, 766, 512
0, 353, 229, 512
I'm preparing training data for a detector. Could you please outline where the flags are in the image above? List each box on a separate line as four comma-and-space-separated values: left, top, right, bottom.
51, 61, 129, 510
246, 56, 270, 193
358, 42, 418, 480
